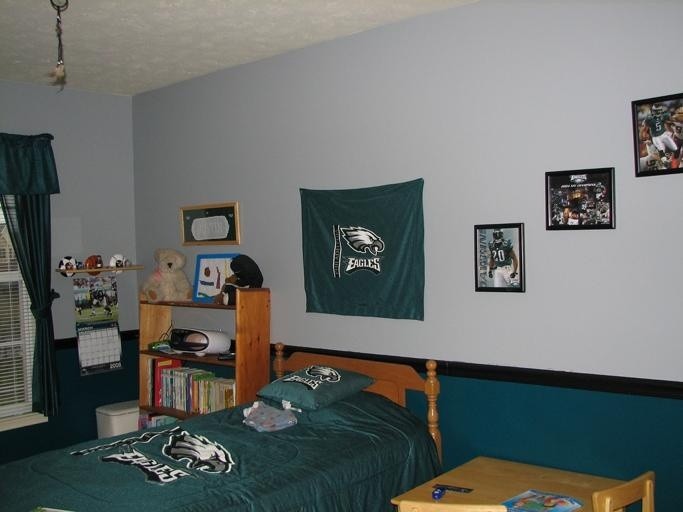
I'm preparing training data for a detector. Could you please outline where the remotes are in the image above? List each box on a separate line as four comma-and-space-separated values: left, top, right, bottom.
218, 354, 234, 360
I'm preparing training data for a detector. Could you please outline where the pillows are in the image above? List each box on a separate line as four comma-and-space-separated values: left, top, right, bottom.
254, 362, 378, 412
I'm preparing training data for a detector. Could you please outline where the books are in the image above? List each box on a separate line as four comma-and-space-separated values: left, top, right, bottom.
145, 354, 234, 415
138, 413, 177, 431
498, 488, 583, 512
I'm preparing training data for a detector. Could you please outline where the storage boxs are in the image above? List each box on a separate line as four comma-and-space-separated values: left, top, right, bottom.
95, 399, 139, 439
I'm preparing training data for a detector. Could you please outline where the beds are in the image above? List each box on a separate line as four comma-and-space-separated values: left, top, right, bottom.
0, 341, 443, 512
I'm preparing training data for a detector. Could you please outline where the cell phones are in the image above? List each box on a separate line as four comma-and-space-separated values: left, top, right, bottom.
432, 486, 445, 499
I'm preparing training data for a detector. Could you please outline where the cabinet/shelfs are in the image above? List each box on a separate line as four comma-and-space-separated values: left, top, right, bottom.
137, 287, 271, 421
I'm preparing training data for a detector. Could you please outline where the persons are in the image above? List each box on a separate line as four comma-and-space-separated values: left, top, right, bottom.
484, 227, 517, 289
73, 277, 117, 318
547, 174, 610, 227
635, 99, 682, 171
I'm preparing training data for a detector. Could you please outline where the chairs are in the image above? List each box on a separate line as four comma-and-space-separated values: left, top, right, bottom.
589, 468, 658, 511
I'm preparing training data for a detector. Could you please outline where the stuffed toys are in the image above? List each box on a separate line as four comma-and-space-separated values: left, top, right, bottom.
220, 253, 263, 307
139, 246, 192, 306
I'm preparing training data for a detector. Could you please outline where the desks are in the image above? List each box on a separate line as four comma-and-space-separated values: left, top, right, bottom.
387, 452, 629, 511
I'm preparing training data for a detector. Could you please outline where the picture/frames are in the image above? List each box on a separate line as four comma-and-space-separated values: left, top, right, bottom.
192, 253, 243, 305
472, 221, 527, 294
629, 91, 683, 179
178, 199, 242, 248
543, 166, 617, 231
